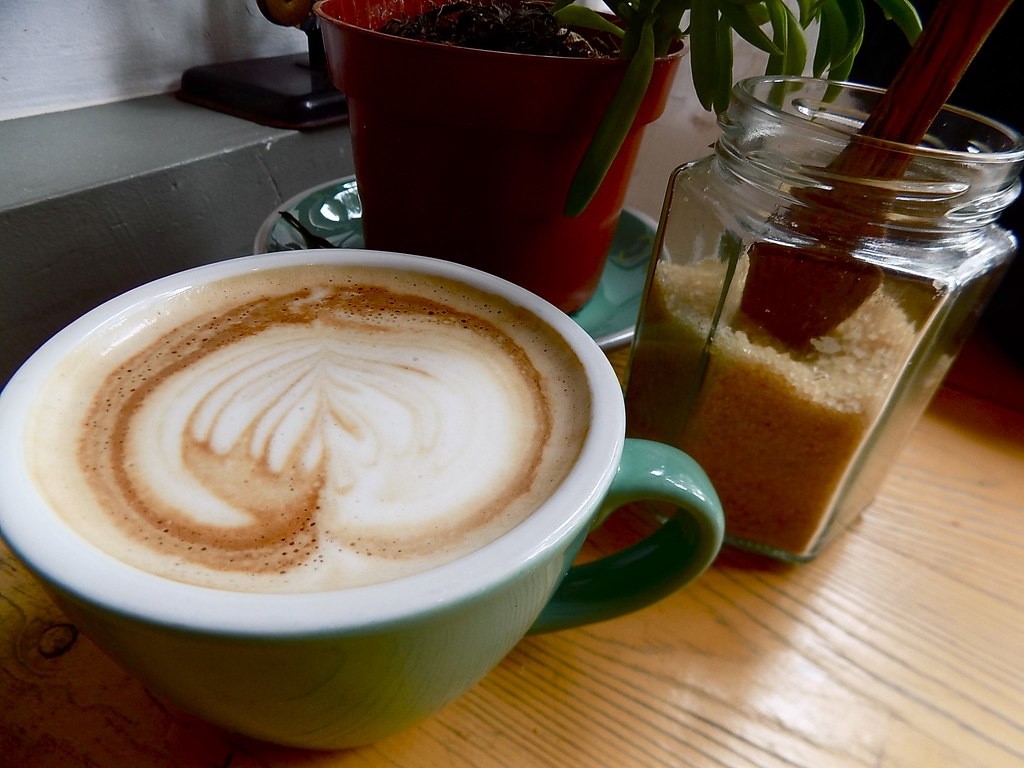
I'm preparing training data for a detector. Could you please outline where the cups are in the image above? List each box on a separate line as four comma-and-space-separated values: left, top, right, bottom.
0, 249, 722, 753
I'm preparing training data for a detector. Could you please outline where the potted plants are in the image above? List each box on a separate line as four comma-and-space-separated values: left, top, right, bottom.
312, 1, 926, 316
